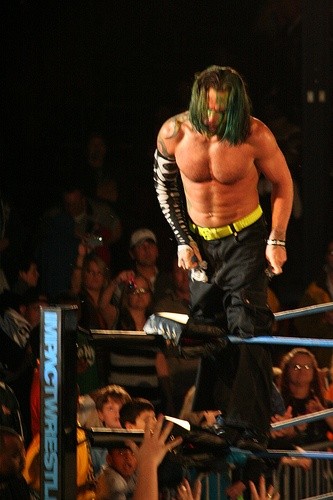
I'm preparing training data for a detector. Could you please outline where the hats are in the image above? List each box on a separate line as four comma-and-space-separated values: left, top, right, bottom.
131, 228, 157, 249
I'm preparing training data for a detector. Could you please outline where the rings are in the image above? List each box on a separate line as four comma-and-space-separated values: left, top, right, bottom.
267, 493, 271, 499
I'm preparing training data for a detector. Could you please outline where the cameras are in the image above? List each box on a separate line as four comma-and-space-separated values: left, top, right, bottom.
88, 234, 103, 247
127, 274, 136, 293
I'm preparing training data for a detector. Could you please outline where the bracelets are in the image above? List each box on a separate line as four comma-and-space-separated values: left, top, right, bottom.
267, 239, 285, 247
74, 264, 84, 271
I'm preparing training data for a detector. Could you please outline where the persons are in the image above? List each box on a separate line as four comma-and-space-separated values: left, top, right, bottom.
152, 66, 294, 452
0, 178, 333, 500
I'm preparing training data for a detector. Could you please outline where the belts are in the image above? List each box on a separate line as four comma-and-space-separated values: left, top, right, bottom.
187, 204, 263, 241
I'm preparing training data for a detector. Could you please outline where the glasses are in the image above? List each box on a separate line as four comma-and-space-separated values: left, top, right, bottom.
325, 249, 333, 255
127, 287, 151, 297
288, 364, 314, 370
